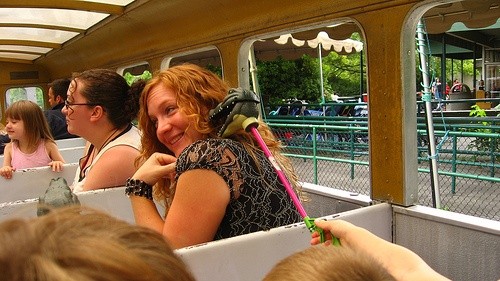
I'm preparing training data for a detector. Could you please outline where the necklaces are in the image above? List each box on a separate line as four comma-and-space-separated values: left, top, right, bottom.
82, 128, 120, 172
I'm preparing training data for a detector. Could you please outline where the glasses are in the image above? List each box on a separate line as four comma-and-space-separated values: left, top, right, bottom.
65, 99, 106, 112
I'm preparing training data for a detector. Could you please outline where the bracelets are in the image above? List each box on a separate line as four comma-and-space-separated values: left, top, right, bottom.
123, 178, 154, 200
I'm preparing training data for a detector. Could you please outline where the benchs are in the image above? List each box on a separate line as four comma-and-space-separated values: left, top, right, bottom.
0, 138, 393, 281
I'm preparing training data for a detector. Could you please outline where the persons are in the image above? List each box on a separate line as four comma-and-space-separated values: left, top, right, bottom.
0, 100, 65, 179
41, 79, 81, 140
265, 99, 312, 141
0, 204, 454, 281
61, 68, 157, 195
432, 77, 462, 110
0, 121, 11, 155
123, 62, 302, 250
